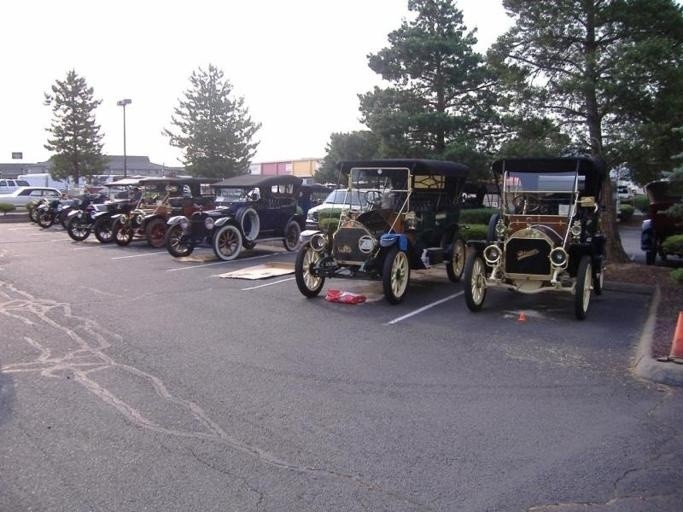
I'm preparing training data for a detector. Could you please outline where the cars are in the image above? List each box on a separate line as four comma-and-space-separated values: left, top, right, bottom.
639, 177, 683, 265
0, 186, 65, 210
616, 184, 636, 205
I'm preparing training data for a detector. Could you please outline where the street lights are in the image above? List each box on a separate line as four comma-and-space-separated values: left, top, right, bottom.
115, 96, 133, 178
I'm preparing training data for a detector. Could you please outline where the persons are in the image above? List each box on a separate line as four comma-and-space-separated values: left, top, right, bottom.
128, 188, 141, 204
57, 194, 68, 202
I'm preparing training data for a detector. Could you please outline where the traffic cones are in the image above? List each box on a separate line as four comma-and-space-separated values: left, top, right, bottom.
654, 309, 682, 364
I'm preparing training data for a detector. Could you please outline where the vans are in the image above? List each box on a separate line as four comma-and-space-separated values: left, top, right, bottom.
0, 178, 31, 194
